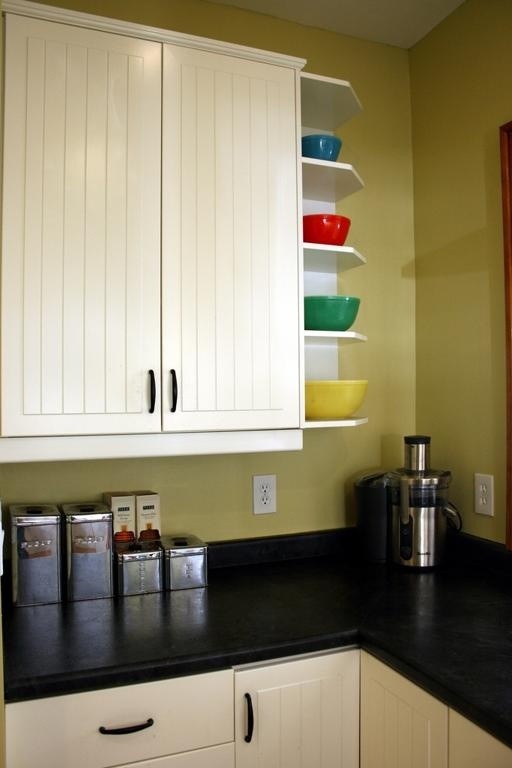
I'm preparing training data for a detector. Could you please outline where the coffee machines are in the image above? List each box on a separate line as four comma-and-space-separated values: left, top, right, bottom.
347, 435, 463, 575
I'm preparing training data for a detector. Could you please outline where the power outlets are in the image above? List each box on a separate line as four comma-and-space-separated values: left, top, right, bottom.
254, 475, 277, 516
474, 473, 494, 516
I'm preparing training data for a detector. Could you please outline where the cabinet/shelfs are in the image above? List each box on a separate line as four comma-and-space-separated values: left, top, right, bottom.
303, 74, 370, 430
236, 635, 512, 768
0, 0, 303, 464
3, 665, 236, 768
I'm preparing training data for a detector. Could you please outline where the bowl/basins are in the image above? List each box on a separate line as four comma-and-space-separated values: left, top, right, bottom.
305, 380, 369, 419
301, 134, 342, 162
303, 294, 361, 331
304, 214, 352, 246
